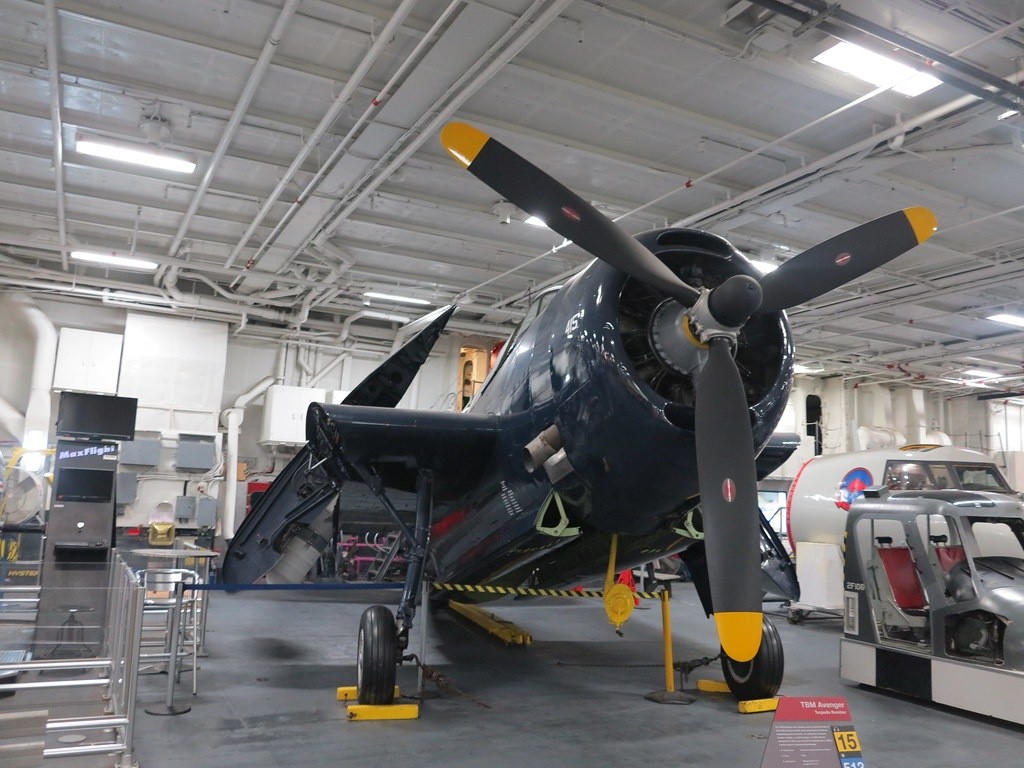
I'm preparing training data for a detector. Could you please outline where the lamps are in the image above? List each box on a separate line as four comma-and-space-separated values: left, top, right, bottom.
812, 34, 944, 97
985, 311, 1024, 330
742, 251, 784, 273
74, 133, 197, 174
960, 367, 1003, 378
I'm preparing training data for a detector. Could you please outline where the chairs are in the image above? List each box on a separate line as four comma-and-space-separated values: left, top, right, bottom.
930, 535, 966, 573
877, 536, 928, 608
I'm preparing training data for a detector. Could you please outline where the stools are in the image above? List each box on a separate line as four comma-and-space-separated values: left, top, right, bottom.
135, 568, 200, 695
39, 606, 96, 675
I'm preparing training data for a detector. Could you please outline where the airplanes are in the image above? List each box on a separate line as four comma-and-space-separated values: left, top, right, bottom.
219, 121, 939, 707
